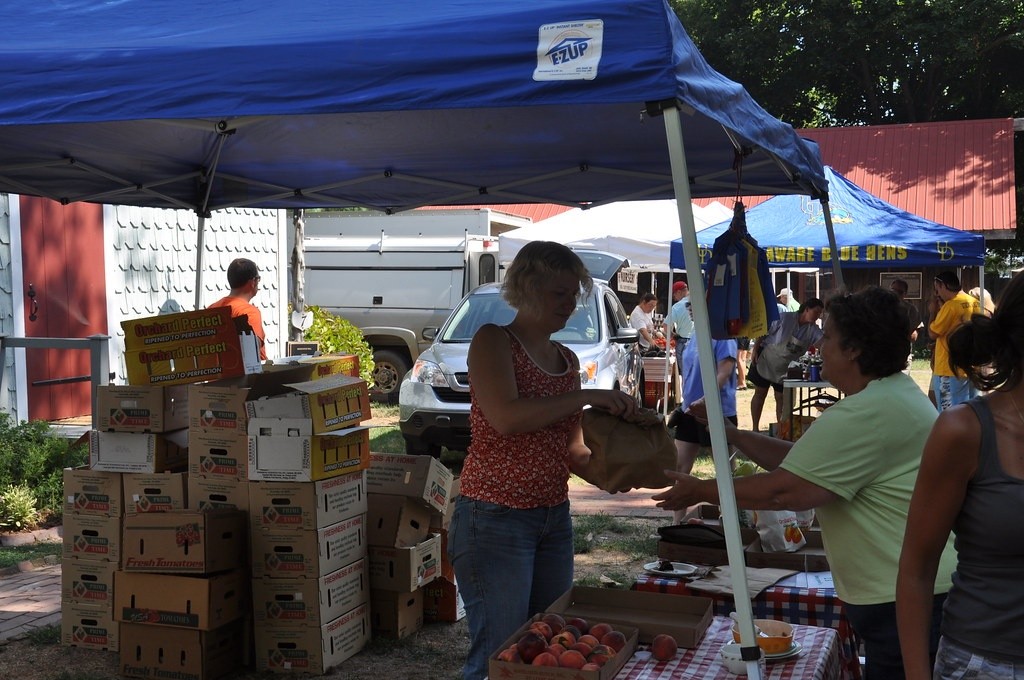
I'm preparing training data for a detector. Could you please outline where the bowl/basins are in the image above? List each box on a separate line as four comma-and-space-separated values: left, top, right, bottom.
720, 644, 766, 674
731, 618, 794, 653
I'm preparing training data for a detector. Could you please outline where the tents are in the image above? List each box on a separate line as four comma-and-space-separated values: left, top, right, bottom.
663, 165, 985, 421
0, 0, 849, 680
496, 200, 820, 419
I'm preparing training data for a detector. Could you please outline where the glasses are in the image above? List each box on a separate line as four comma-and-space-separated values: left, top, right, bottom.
934, 276, 944, 283
249, 275, 261, 281
891, 288, 906, 295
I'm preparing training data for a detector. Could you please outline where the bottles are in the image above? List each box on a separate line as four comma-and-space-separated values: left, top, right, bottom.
810, 363, 819, 382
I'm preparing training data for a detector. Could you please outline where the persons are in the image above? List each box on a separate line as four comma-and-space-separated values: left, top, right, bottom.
746, 298, 824, 432
208, 258, 268, 361
891, 280, 925, 375
650, 292, 738, 540
663, 281, 694, 375
756, 288, 801, 347
927, 272, 995, 414
447, 242, 639, 680
736, 336, 751, 390
630, 293, 660, 349
652, 285, 960, 680
896, 271, 1024, 680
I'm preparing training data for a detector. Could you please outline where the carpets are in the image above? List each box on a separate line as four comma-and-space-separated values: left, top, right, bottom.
49, 415, 92, 426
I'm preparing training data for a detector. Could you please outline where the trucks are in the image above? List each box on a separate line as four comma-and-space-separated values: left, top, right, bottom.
304, 236, 512, 404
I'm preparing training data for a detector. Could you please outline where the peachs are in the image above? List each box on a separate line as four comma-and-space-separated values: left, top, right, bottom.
496, 613, 626, 672
651, 634, 677, 660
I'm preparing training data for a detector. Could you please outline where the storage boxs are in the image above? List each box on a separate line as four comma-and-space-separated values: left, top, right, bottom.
656, 506, 830, 571
487, 613, 639, 680
61, 305, 470, 680
545, 585, 713, 649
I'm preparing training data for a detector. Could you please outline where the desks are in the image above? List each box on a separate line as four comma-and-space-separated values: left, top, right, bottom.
642, 356, 677, 414
783, 378, 846, 441
615, 559, 864, 680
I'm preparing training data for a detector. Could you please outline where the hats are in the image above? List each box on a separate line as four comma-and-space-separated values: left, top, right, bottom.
673, 281, 687, 297
777, 288, 793, 297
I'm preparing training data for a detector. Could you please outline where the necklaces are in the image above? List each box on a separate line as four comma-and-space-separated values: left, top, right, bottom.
1009, 391, 1024, 423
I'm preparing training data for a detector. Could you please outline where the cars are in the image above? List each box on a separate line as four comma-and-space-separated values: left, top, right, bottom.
400, 248, 646, 459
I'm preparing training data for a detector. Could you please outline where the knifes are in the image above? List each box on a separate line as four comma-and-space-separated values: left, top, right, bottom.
729, 611, 770, 638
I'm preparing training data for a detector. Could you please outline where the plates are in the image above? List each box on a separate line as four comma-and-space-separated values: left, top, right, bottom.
644, 561, 698, 576
764, 641, 803, 661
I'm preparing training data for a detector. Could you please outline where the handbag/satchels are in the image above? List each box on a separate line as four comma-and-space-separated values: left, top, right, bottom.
668, 406, 682, 428
581, 406, 678, 491
